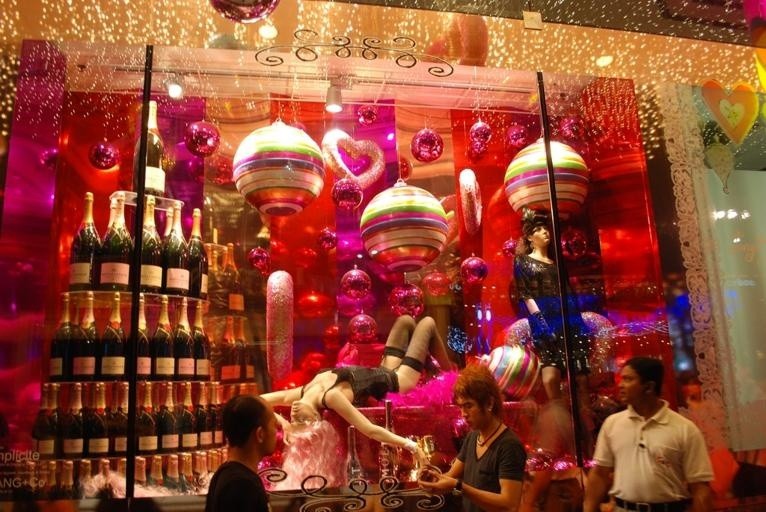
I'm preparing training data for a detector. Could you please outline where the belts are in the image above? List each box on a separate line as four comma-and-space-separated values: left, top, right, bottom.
613, 496, 692, 512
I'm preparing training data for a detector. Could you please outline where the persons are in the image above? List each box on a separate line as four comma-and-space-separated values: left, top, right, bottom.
417, 359, 529, 512
204, 393, 277, 512
583, 356, 714, 512
514, 213, 598, 459
259, 315, 453, 467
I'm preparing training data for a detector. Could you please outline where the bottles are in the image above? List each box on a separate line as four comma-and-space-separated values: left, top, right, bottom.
178, 382, 199, 452
98, 291, 127, 380
31, 380, 60, 461
161, 202, 173, 245
83, 380, 110, 458
174, 296, 194, 383
26, 458, 126, 499
137, 292, 152, 381
69, 291, 99, 382
209, 249, 229, 316
161, 199, 189, 295
217, 311, 242, 384
93, 189, 133, 293
67, 190, 102, 290
344, 424, 363, 484
236, 317, 257, 383
193, 297, 211, 382
47, 290, 74, 382
135, 381, 160, 455
157, 381, 181, 455
47, 383, 60, 431
139, 193, 163, 293
134, 450, 228, 493
133, 99, 167, 196
107, 380, 128, 457
59, 383, 84, 461
378, 398, 401, 484
149, 295, 174, 380
196, 380, 215, 451
210, 380, 228, 448
223, 241, 246, 316
72, 298, 81, 325
188, 208, 210, 301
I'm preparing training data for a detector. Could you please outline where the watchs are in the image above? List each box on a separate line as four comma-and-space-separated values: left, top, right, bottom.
452, 478, 464, 498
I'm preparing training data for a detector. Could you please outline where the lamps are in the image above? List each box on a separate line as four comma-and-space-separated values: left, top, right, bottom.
163, 77, 183, 98
325, 87, 343, 113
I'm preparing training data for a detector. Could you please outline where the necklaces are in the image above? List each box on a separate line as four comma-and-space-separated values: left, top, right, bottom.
476, 417, 505, 447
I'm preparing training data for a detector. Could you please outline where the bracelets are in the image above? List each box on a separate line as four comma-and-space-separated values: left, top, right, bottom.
406, 439, 418, 455
